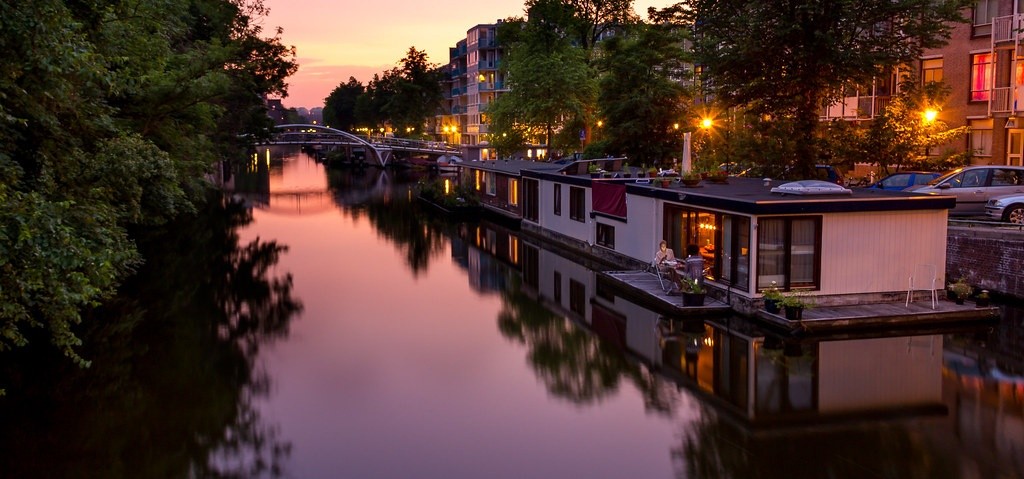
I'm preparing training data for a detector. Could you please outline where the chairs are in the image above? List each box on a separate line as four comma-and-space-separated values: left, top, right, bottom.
651, 257, 679, 291
905, 263, 939, 310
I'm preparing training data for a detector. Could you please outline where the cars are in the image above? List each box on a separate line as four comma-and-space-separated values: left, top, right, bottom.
986, 192, 1024, 228
868, 170, 960, 193
899, 164, 1024, 217
609, 159, 846, 187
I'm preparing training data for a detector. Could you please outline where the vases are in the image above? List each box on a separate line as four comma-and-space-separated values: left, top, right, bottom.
682, 295, 705, 306
765, 300, 782, 313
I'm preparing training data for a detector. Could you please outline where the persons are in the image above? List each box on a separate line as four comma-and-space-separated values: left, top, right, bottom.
684, 245, 704, 284
992, 170, 1010, 186
656, 240, 687, 292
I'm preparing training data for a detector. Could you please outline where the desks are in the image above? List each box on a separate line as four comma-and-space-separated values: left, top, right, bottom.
660, 264, 685, 295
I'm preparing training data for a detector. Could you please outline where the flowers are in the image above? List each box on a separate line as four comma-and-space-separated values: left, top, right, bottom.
762, 280, 784, 301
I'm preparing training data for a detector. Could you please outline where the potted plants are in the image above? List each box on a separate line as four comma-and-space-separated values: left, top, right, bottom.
775, 289, 816, 321
974, 292, 990, 308
947, 279, 974, 305
680, 278, 706, 294
589, 162, 729, 189
